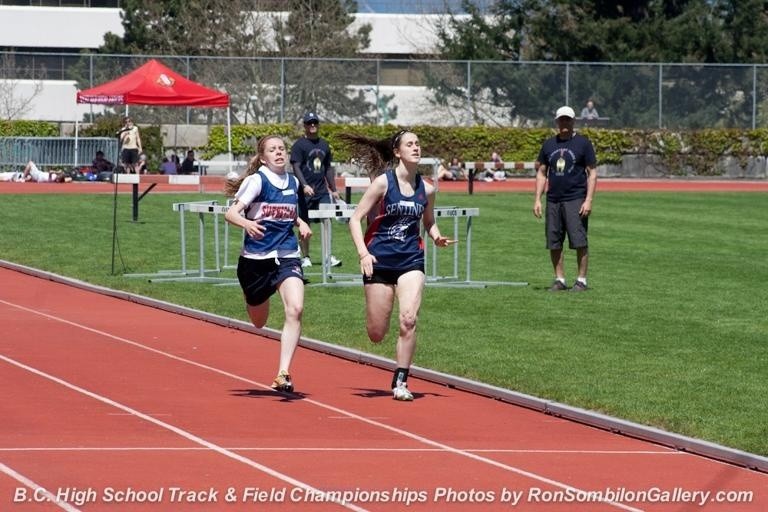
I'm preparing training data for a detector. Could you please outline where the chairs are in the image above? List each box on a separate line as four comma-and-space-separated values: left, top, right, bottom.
301, 257, 313, 267
272, 372, 294, 393
548, 280, 566, 291
569, 281, 586, 291
16, 178, 25, 183
393, 382, 414, 400
331, 255, 343, 267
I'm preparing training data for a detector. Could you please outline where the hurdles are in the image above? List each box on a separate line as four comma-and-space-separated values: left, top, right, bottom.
460, 161, 543, 196
124, 199, 529, 287
113, 174, 249, 223
334, 176, 438, 220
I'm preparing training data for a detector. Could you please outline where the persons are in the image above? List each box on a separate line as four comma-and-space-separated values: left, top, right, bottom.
429, 152, 504, 182
224, 136, 313, 393
533, 106, 597, 292
290, 113, 342, 268
333, 130, 459, 401
0, 117, 198, 183
581, 101, 599, 120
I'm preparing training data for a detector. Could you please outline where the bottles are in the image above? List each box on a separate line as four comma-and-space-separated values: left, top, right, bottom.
553, 106, 575, 120
304, 110, 319, 123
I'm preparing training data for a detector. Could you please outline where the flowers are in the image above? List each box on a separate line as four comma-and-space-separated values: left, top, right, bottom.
115, 126, 133, 139
